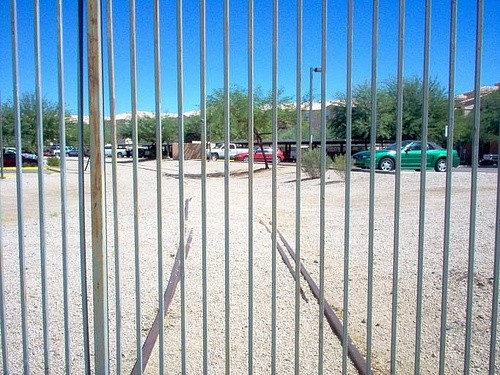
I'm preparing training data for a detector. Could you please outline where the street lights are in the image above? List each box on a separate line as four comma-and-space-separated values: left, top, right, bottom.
309, 66, 323, 155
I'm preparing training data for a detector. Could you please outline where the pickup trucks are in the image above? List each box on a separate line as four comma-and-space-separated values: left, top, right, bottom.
205, 143, 249, 161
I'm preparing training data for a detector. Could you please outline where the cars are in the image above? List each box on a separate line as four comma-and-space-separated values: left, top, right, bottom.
455, 139, 499, 167
1, 138, 173, 168
353, 139, 460, 173
235, 146, 284, 165
